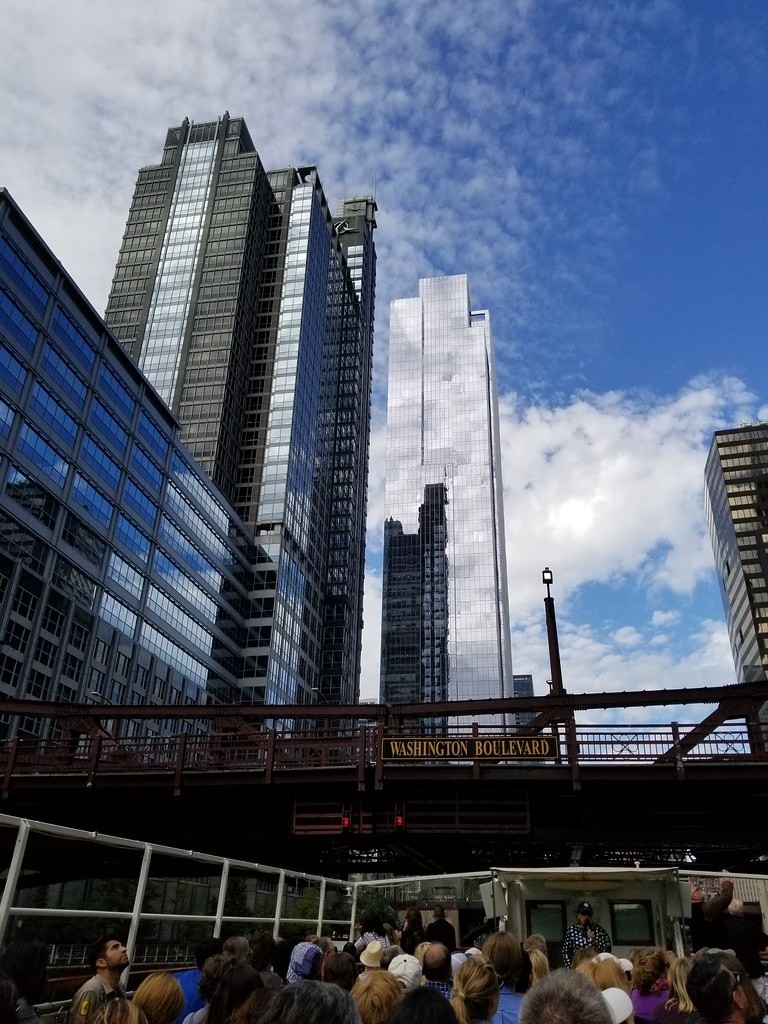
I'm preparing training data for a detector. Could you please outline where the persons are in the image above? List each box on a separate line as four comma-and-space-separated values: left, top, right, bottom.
0, 899, 768, 1024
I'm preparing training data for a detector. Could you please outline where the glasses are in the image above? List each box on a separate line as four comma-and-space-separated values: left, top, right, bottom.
580, 911, 593, 916
724, 971, 741, 995
692, 887, 702, 896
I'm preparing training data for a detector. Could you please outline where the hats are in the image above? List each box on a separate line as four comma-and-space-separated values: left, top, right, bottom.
447, 953, 473, 981
600, 987, 634, 1024
388, 953, 423, 993
591, 953, 633, 973
360, 941, 386, 967
578, 902, 592, 912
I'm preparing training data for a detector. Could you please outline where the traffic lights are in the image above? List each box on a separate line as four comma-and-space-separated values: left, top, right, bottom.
395, 801, 405, 832
342, 803, 350, 832
